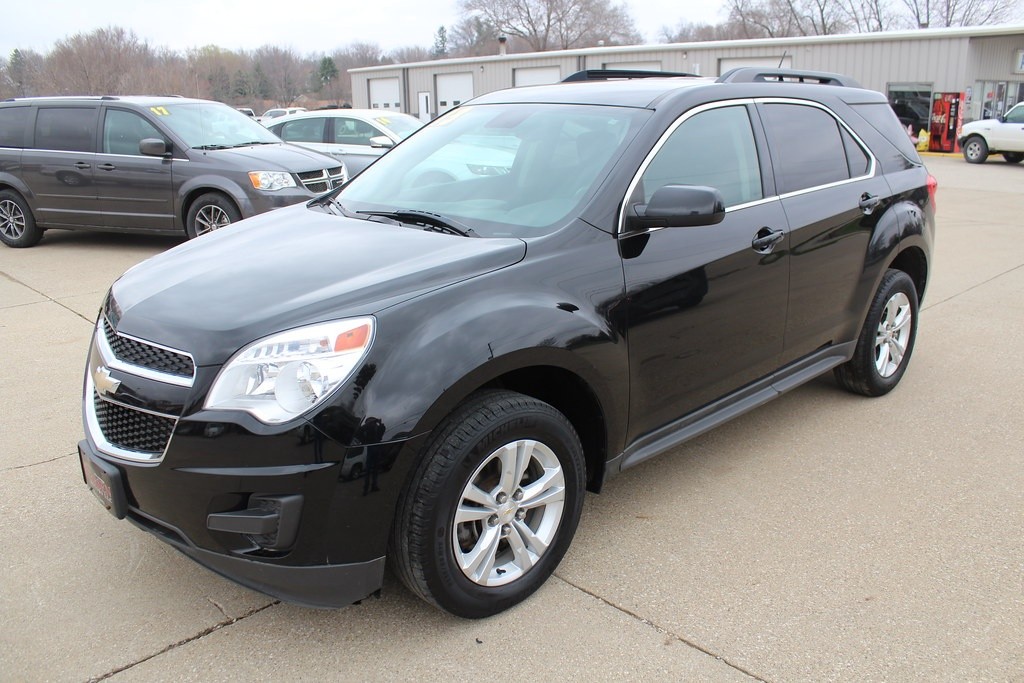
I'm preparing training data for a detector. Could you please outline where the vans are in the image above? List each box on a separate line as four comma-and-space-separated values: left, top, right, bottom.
0, 95, 350, 247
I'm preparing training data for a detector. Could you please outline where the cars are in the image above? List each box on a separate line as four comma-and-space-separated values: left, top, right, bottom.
957, 100, 1024, 163
235, 106, 515, 204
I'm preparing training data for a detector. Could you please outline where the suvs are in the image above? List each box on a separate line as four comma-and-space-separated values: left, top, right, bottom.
890, 97, 929, 136
79, 69, 935, 622
